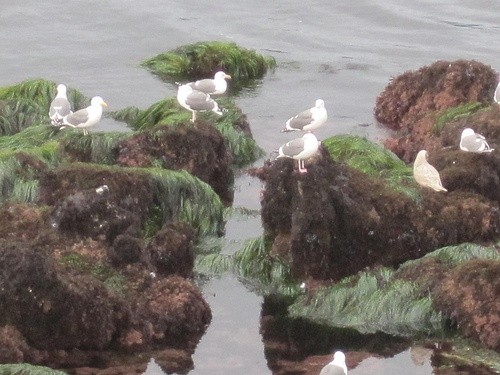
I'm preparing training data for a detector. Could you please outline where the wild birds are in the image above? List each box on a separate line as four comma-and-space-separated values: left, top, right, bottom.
49, 84, 72, 128
280, 99, 328, 133
176, 84, 228, 123
187, 71, 231, 96
319, 351, 349, 375
275, 132, 320, 172
413, 149, 449, 192
459, 128, 496, 153
59, 96, 107, 137
